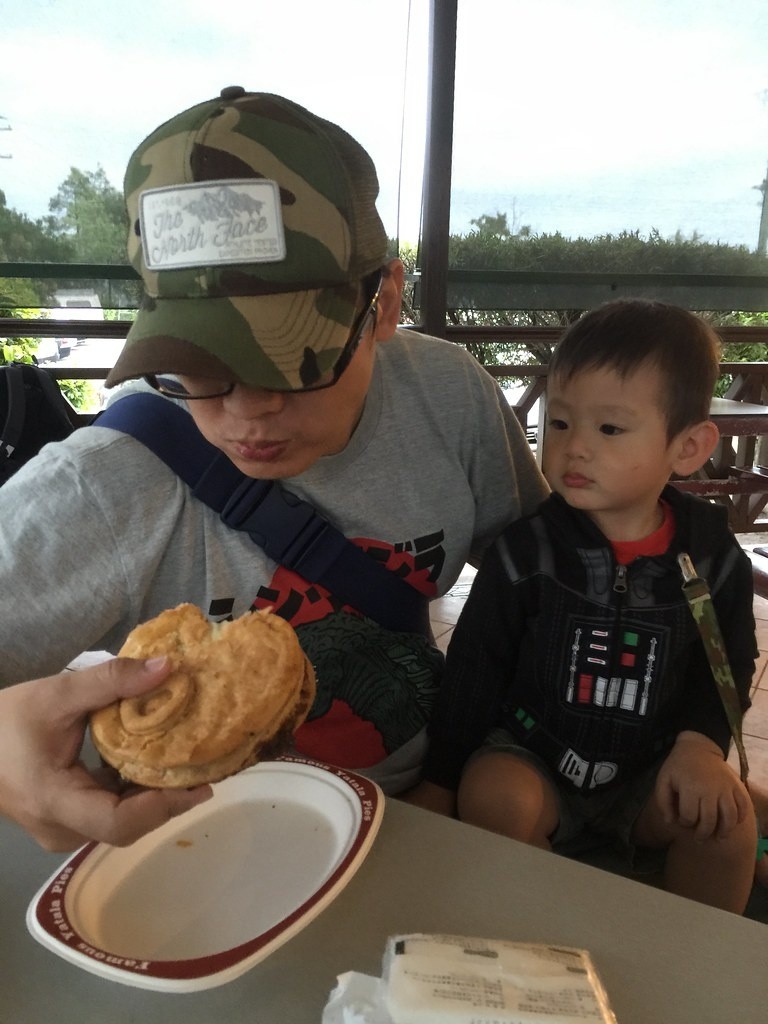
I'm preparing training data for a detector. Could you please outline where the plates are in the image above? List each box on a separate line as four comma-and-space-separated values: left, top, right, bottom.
26, 755, 386, 994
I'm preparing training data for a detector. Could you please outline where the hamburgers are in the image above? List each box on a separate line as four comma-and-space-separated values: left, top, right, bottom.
90, 603, 315, 789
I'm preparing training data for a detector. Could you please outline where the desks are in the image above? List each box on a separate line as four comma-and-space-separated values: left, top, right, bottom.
0, 756, 768, 1024
662, 393, 768, 537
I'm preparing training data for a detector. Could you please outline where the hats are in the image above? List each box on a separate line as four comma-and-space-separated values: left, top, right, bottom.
104, 86, 388, 394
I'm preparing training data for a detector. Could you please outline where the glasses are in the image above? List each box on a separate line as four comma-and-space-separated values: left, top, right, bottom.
144, 271, 383, 400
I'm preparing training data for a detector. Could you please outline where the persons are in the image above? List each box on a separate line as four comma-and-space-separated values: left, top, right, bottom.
403, 302, 759, 917
1, 82, 552, 852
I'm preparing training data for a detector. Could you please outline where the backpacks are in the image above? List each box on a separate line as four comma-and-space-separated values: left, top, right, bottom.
0, 362, 86, 487
